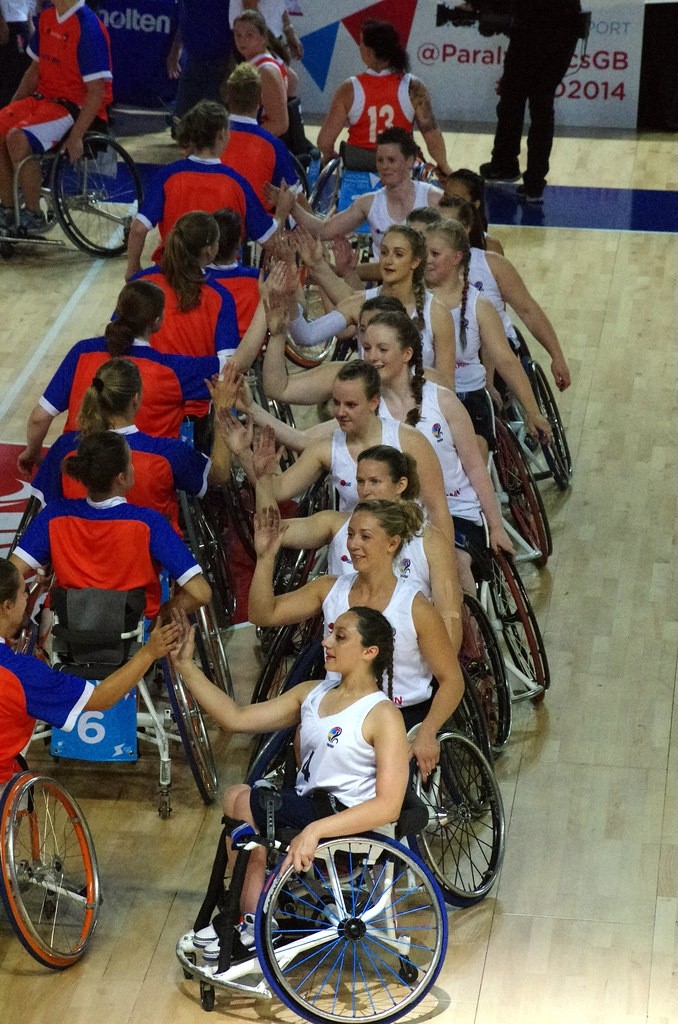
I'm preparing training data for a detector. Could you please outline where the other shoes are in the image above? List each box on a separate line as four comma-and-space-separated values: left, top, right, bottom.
2, 208, 48, 238
515, 182, 544, 206
191, 921, 242, 948
0, 205, 21, 228
480, 154, 521, 182
204, 913, 282, 961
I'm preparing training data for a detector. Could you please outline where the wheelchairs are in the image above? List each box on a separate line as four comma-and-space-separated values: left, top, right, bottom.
0, 105, 143, 259
0, 96, 575, 1023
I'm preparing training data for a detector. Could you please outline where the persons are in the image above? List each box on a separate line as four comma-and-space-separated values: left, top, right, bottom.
17, 287, 517, 656
261, 126, 570, 465
110, 207, 269, 424
125, 11, 300, 282
456, 0, 582, 202
165, 424, 465, 962
0, 557, 180, 788
0, 0, 304, 230
317, 16, 453, 176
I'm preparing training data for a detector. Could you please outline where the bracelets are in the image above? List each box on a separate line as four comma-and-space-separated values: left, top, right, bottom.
283, 26, 294, 32
